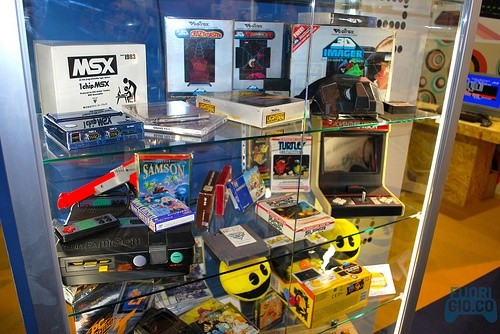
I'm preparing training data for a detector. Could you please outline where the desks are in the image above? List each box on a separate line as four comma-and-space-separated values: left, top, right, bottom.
401, 104, 500, 208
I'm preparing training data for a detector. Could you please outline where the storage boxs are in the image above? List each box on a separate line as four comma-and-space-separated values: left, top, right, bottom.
32, 13, 406, 334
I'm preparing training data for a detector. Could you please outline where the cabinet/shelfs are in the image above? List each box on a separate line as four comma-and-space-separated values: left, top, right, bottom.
0, 0, 482, 334
